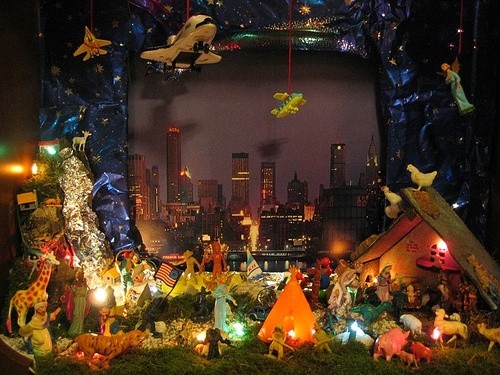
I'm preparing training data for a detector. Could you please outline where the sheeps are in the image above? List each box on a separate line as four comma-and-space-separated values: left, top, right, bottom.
477, 323, 500, 352
434, 309, 468, 349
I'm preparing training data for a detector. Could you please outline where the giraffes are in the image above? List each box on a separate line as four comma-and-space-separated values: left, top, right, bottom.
6, 247, 60, 341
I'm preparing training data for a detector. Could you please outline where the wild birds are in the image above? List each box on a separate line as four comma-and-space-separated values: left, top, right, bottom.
381, 185, 402, 210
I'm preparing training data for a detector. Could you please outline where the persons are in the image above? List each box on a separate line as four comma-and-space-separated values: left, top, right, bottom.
179, 241, 499, 362
27, 302, 61, 357
441, 63, 474, 116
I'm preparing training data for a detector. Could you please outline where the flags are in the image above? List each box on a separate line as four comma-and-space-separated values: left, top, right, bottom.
153, 261, 181, 287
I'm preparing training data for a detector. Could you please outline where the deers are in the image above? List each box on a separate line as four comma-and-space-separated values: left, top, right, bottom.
72, 130, 92, 152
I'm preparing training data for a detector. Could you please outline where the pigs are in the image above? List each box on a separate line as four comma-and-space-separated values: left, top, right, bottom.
409, 340, 431, 364
373, 327, 410, 362
396, 350, 418, 368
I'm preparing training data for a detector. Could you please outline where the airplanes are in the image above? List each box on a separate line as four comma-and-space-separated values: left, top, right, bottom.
271, 90, 307, 118
141, 14, 222, 80
72, 26, 111, 62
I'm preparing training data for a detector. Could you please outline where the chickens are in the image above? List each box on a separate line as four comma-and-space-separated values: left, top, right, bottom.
407, 164, 437, 191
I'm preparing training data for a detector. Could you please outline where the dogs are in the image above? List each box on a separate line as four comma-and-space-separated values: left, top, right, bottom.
399, 314, 422, 336
